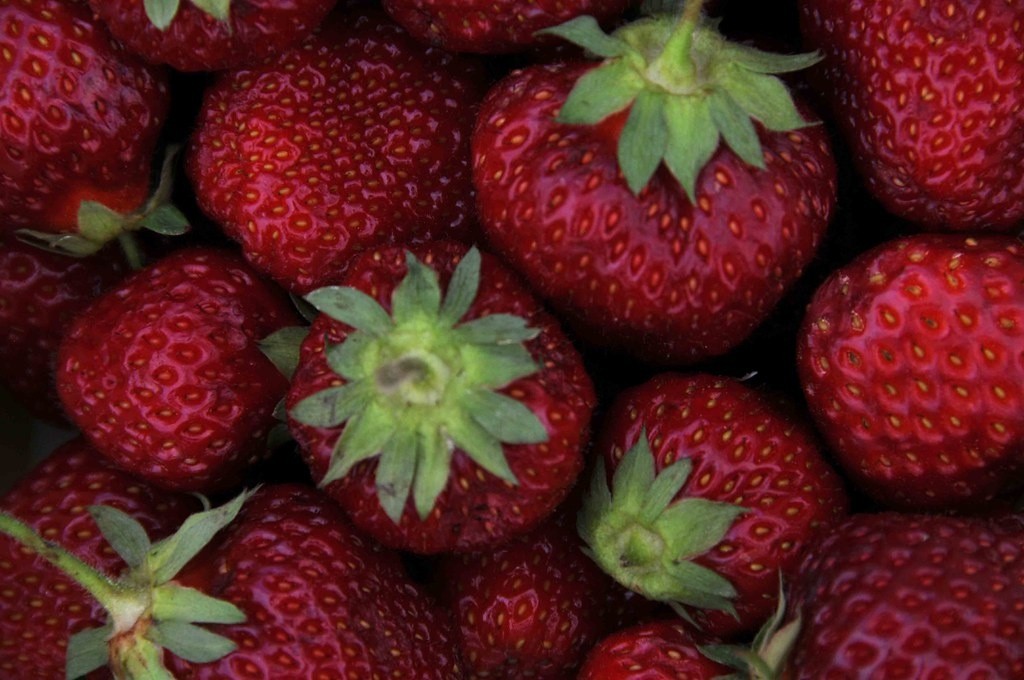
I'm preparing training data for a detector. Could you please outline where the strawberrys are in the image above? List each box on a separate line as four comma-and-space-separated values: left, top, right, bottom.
0, 0, 1024, 680
